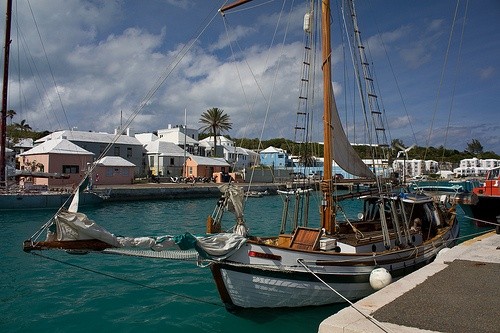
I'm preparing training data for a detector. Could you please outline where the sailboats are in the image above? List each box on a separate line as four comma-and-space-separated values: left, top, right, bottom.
21, 0, 479, 318
